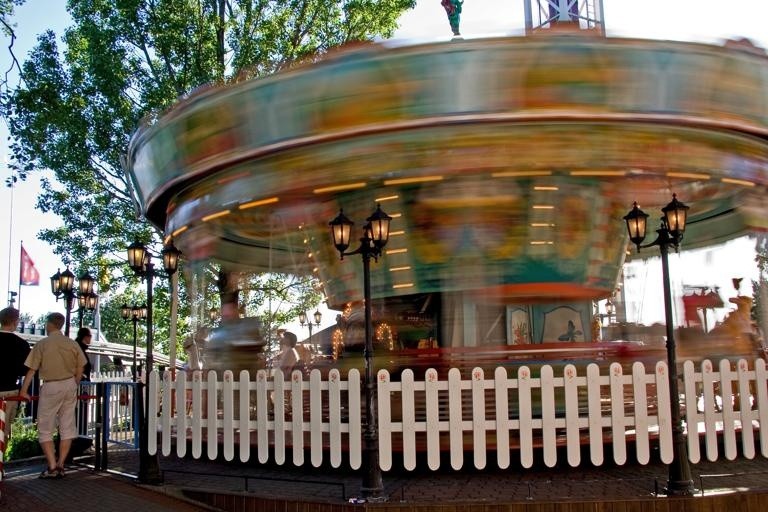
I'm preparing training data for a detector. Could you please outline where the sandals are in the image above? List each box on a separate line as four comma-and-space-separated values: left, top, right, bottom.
39, 466, 66, 478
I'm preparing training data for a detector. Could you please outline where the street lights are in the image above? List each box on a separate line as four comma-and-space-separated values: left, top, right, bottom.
120, 301, 150, 430
124, 235, 181, 485
298, 309, 321, 344
594, 301, 613, 343
49, 267, 93, 343
327, 201, 392, 501
62, 291, 99, 332
621, 191, 701, 500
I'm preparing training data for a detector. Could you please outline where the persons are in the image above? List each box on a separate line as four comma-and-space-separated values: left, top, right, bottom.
18, 312, 89, 480
75, 327, 92, 435
266, 331, 302, 416
1, 306, 32, 479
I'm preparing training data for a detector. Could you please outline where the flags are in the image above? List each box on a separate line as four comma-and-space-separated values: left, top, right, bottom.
20, 248, 42, 286
